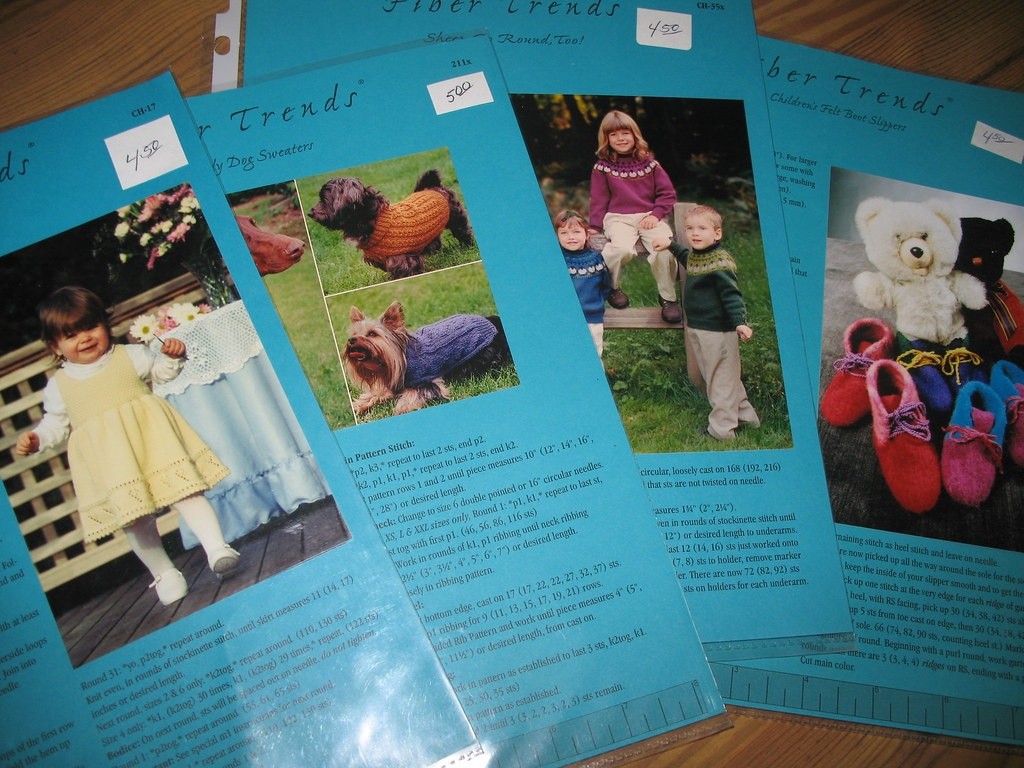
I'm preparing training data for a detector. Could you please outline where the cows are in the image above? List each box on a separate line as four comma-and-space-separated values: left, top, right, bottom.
231, 211, 306, 286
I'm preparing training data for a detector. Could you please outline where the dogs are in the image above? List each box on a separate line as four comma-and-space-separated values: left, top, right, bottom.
306, 165, 471, 277
337, 298, 514, 415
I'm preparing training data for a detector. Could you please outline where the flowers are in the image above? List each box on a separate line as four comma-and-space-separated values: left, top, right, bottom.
130, 303, 213, 343
112, 182, 240, 308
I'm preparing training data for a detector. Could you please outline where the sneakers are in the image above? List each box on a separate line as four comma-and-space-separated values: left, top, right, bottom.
658, 294, 682, 323
607, 286, 629, 308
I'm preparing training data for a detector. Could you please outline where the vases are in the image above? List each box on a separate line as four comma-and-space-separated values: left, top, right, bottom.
177, 246, 240, 307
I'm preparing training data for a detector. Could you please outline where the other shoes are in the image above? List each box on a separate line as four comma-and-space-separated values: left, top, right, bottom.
148, 569, 188, 606
940, 381, 1007, 505
207, 543, 241, 580
988, 360, 1024, 467
867, 358, 941, 513
821, 316, 895, 428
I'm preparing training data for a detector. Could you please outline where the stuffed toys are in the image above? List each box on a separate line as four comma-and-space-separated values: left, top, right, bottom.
856, 198, 1024, 411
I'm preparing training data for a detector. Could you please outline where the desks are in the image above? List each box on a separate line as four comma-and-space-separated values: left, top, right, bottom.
151, 297, 330, 546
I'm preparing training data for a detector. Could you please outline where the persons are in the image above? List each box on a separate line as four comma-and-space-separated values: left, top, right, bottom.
16, 285, 240, 606
589, 110, 681, 324
552, 209, 609, 373
653, 205, 762, 440
